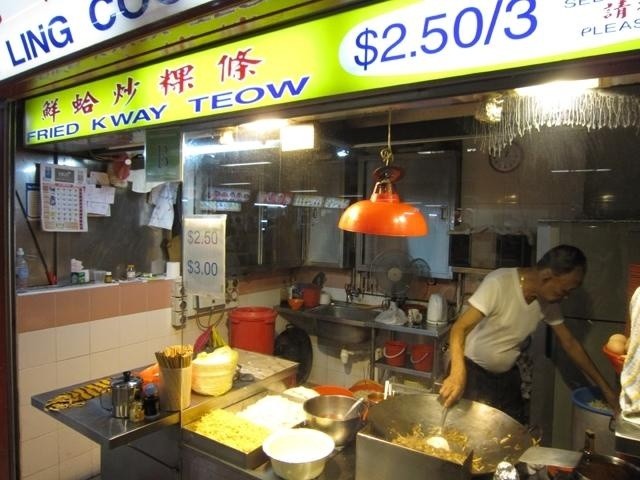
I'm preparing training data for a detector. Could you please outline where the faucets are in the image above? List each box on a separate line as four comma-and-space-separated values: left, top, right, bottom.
346, 286, 360, 303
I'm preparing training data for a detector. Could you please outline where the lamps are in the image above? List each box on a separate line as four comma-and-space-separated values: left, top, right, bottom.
337, 107, 429, 239
87, 138, 133, 179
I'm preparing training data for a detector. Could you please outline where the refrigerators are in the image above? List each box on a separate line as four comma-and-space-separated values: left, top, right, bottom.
535, 216, 639, 453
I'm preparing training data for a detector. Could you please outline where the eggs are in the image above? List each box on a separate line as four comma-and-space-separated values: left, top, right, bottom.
607, 334, 631, 354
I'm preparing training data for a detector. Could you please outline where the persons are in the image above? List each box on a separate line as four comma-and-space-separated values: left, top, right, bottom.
438, 244, 622, 426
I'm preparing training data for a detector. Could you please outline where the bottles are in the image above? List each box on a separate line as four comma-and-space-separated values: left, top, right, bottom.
16, 248, 29, 293
126, 263, 136, 282
105, 271, 113, 283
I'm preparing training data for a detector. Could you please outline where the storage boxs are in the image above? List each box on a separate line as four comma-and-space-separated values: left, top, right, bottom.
388, 372, 444, 395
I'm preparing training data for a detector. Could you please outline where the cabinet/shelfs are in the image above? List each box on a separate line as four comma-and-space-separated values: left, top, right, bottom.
369, 330, 450, 395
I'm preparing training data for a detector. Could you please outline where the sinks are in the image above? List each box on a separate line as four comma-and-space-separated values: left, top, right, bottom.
303, 303, 381, 343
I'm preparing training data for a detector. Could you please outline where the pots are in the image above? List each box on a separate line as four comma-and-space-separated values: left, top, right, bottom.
367, 376, 544, 474
274, 322, 314, 386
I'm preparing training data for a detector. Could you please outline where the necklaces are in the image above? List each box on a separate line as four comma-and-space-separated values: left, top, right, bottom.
519, 267, 526, 289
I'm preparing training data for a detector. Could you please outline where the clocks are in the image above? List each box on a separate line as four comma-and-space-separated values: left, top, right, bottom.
489, 140, 524, 172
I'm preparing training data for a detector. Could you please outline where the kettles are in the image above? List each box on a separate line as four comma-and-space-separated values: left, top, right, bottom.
426, 290, 450, 326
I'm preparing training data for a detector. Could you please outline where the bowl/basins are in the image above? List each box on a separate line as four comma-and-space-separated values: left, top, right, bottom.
301, 391, 368, 441
288, 298, 305, 312
262, 427, 336, 480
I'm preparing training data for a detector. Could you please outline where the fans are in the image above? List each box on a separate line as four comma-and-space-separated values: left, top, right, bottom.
369, 250, 419, 324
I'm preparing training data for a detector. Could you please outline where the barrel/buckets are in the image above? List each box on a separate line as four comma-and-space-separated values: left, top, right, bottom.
572, 385, 618, 456
409, 343, 434, 371
225, 305, 278, 357
382, 340, 409, 366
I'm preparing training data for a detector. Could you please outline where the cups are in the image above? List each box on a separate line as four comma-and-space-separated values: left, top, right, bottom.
407, 308, 423, 324
99, 370, 147, 421
